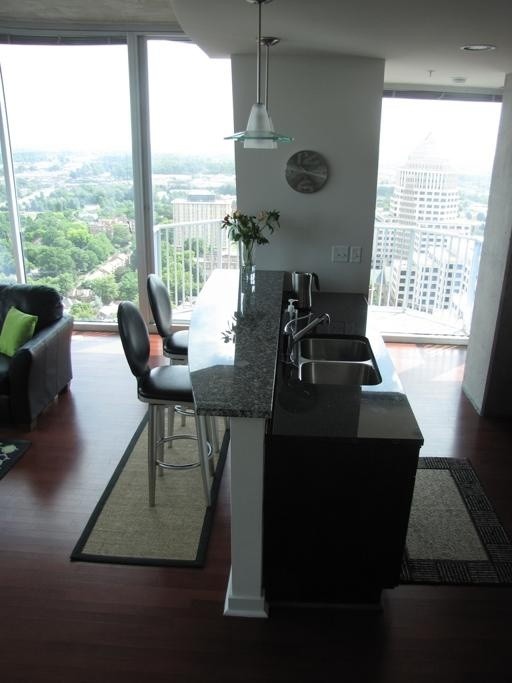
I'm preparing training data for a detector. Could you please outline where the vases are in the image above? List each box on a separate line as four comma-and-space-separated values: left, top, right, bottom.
241, 239, 256, 272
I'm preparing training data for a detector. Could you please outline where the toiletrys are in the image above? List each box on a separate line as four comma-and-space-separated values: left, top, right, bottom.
284, 299, 299, 333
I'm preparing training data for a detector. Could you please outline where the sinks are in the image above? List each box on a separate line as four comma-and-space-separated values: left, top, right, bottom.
297, 333, 375, 362
298, 357, 382, 385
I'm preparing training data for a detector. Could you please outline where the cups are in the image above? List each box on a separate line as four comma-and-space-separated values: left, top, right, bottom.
292, 270, 314, 310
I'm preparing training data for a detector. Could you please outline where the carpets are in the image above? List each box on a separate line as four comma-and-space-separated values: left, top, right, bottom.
0, 437, 32, 480
398, 457, 512, 586
68, 403, 229, 569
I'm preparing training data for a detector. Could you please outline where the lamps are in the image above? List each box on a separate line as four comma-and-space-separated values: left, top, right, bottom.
224, 0, 295, 149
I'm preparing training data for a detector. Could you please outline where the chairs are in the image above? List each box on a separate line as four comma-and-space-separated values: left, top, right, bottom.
147, 273, 219, 453
117, 300, 211, 508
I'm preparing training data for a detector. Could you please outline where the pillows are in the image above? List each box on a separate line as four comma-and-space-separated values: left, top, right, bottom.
0, 305, 38, 358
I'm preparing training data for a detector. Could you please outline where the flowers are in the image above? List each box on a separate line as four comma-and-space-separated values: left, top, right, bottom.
220, 207, 280, 269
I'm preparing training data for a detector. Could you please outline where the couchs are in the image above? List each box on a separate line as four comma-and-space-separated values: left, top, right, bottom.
0, 284, 73, 433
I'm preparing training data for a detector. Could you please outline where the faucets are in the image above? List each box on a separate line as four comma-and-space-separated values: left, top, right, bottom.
281, 313, 331, 364
